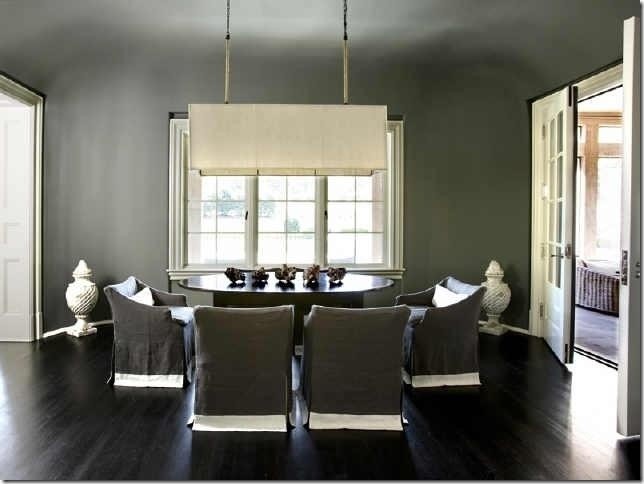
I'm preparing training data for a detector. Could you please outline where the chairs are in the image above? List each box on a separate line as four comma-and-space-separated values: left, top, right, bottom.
188, 307, 293, 436
297, 304, 406, 432
395, 275, 483, 389
102, 273, 193, 391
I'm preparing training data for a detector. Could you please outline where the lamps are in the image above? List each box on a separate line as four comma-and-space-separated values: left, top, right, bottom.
187, 2, 388, 176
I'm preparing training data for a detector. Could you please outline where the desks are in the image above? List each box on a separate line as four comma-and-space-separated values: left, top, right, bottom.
177, 270, 396, 366
